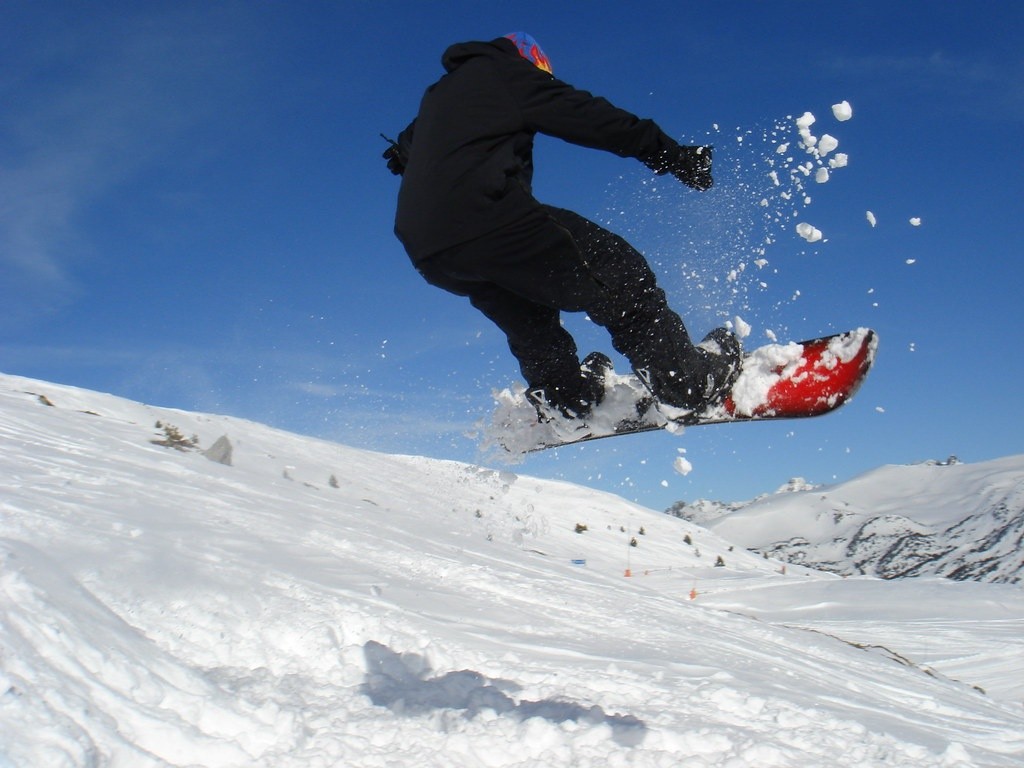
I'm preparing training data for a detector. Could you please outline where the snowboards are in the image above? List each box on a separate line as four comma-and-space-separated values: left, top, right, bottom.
499, 328, 876, 453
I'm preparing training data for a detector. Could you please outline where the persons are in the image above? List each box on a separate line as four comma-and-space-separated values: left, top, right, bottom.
378, 31, 743, 428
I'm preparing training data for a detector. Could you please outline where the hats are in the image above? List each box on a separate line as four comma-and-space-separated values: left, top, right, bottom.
502, 32, 552, 75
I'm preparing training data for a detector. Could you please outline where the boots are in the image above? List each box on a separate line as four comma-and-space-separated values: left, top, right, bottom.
635, 326, 743, 425
524, 352, 613, 425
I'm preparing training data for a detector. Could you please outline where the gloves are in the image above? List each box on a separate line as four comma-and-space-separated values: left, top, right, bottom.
656, 145, 713, 193
379, 133, 405, 176
